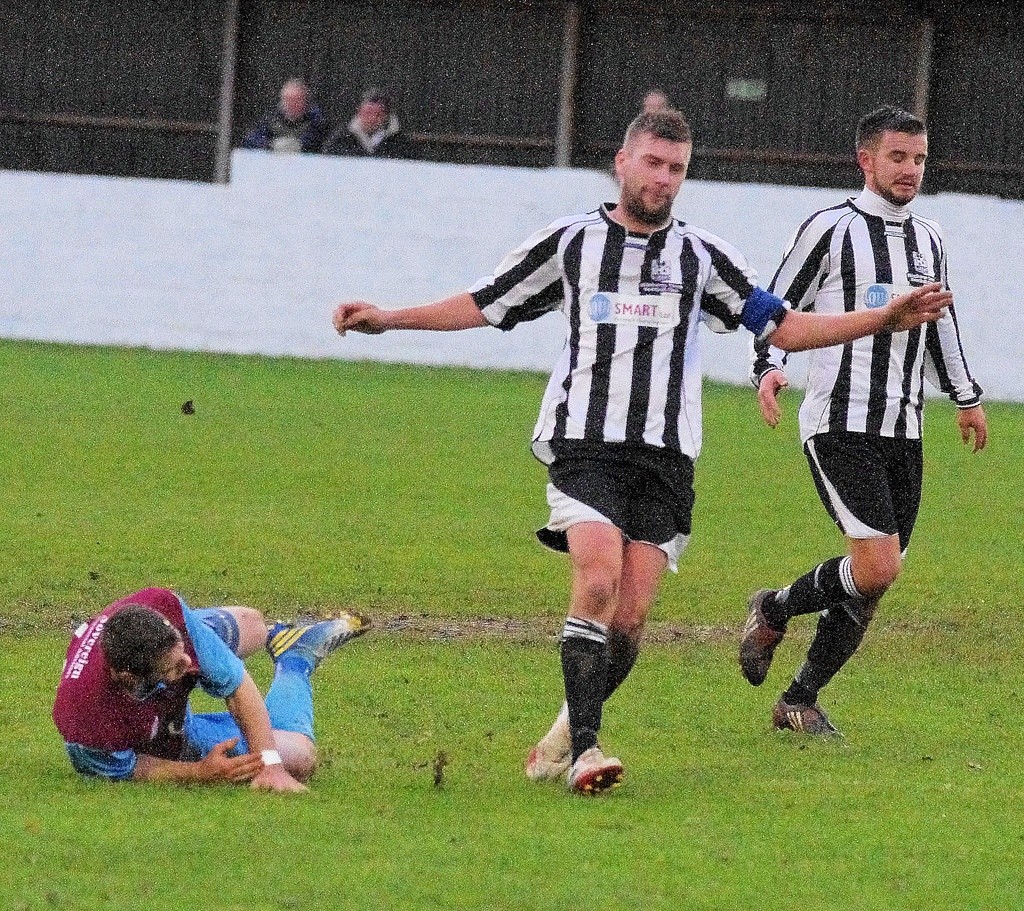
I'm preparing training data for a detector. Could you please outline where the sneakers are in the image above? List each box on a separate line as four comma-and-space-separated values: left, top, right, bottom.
740, 588, 786, 686
267, 613, 372, 662
568, 748, 625, 794
525, 750, 572, 780
773, 698, 846, 737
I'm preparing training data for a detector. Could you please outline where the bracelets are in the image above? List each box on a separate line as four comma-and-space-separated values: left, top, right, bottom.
261, 750, 282, 766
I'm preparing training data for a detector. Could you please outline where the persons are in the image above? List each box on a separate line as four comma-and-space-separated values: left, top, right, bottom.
334, 112, 951, 794
644, 90, 672, 111
740, 104, 989, 738
235, 80, 328, 154
324, 89, 415, 160
52, 588, 373, 793
